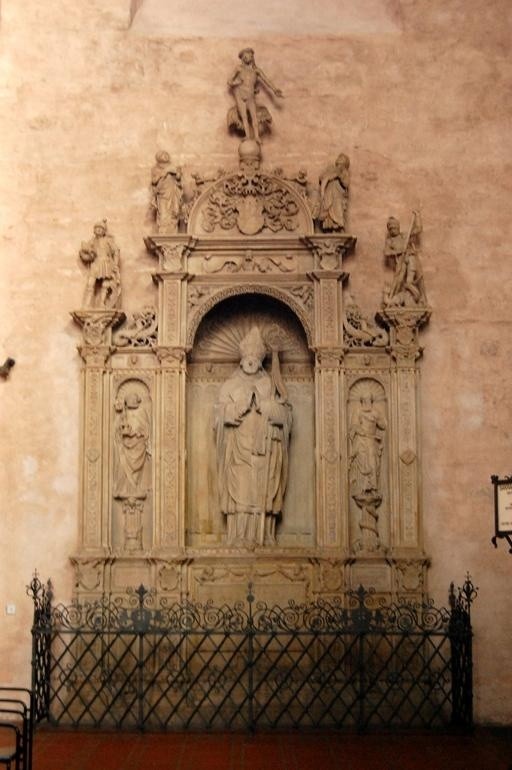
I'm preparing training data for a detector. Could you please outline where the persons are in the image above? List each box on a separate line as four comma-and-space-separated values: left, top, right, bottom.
212, 322, 291, 545
113, 392, 149, 499
349, 390, 386, 498
81, 219, 121, 309
227, 47, 284, 144
384, 210, 423, 307
151, 150, 183, 233
319, 153, 352, 229
